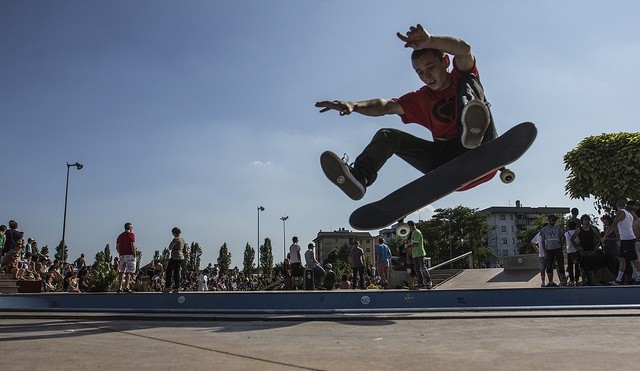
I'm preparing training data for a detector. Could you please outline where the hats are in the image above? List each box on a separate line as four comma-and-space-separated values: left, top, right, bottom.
549, 216, 560, 219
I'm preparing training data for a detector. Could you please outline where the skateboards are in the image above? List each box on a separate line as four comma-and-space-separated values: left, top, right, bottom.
411, 285, 432, 290
349, 121, 538, 237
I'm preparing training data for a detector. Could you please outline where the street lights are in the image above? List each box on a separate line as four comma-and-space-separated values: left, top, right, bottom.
61, 161, 84, 277
440, 218, 453, 270
280, 216, 290, 259
258, 205, 265, 282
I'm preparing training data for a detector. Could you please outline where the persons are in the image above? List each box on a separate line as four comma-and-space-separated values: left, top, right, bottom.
185, 266, 197, 292
195, 262, 283, 292
400, 221, 433, 289
601, 200, 640, 285
570, 213, 601, 288
35, 252, 87, 294
567, 208, 581, 228
599, 215, 616, 285
473, 261, 502, 268
375, 235, 392, 289
348, 273, 354, 281
402, 231, 418, 288
564, 222, 580, 288
305, 241, 326, 290
625, 207, 639, 282
149, 261, 166, 293
162, 227, 189, 292
112, 256, 119, 275
116, 222, 137, 293
350, 240, 367, 289
340, 273, 350, 288
1, 219, 37, 281
284, 236, 306, 289
540, 215, 566, 287
314, 24, 498, 202
530, 223, 552, 288
324, 263, 335, 290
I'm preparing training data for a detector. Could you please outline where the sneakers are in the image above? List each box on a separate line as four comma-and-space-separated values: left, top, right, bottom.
172, 289, 178, 293
123, 288, 131, 292
460, 100, 491, 150
117, 289, 122, 293
318, 285, 327, 289
321, 150, 366, 201
161, 288, 171, 293
541, 283, 545, 287
415, 284, 424, 287
426, 281, 432, 288
610, 280, 623, 287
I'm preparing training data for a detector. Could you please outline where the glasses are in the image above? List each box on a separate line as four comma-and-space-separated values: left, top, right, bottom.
586, 219, 591, 222
